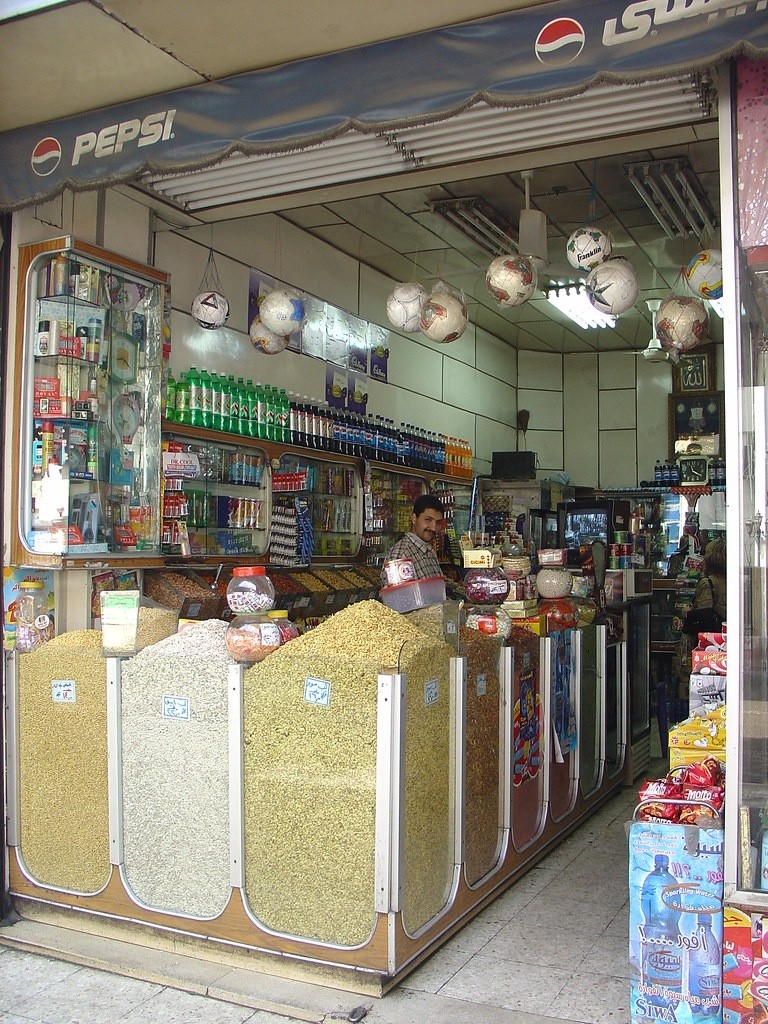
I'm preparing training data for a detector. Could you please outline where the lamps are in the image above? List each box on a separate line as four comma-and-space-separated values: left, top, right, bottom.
422, 154, 720, 331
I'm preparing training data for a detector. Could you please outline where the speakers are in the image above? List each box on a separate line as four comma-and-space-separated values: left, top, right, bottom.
492, 451, 536, 479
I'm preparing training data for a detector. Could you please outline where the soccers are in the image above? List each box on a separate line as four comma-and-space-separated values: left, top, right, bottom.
191, 291, 230, 330
486, 254, 538, 307
685, 248, 723, 300
585, 259, 639, 315
386, 283, 429, 333
566, 226, 612, 273
655, 298, 709, 351
249, 289, 305, 355
419, 293, 468, 343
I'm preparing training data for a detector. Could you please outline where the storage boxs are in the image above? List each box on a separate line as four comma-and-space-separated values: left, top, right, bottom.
4, 528, 724, 1024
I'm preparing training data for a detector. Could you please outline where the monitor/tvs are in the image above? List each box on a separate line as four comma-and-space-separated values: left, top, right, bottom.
557, 499, 630, 566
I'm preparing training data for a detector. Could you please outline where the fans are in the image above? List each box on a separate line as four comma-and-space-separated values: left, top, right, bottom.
570, 297, 714, 369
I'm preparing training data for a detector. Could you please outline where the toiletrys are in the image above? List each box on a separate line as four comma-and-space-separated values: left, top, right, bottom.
37, 256, 101, 480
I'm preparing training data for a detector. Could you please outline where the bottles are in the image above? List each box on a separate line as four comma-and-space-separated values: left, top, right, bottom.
628, 512, 639, 534
186, 488, 211, 527
164, 367, 191, 425
331, 404, 410, 466
288, 389, 334, 452
707, 458, 717, 486
639, 854, 684, 1011
654, 459, 679, 486
518, 653, 537, 741
189, 364, 291, 445
411, 425, 473, 479
717, 458, 726, 486
687, 912, 722, 1017
761, 829, 768, 890
551, 630, 572, 734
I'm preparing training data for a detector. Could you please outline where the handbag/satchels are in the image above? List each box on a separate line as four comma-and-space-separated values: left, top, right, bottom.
681, 576, 723, 634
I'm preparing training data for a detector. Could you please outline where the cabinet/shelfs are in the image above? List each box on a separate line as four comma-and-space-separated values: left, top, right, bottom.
11, 233, 477, 572
652, 579, 680, 716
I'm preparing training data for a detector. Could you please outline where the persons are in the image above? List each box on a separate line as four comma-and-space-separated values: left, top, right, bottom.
694, 530, 726, 630
379, 495, 445, 588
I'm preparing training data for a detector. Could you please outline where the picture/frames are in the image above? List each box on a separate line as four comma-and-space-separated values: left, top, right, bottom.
665, 346, 725, 464
